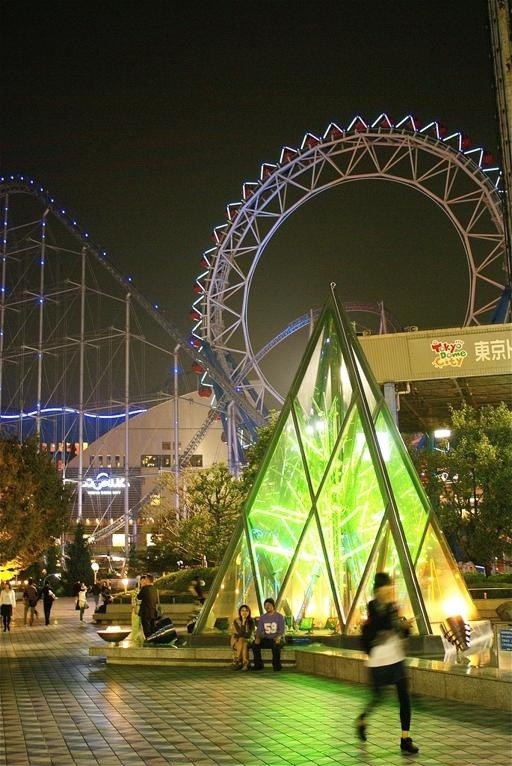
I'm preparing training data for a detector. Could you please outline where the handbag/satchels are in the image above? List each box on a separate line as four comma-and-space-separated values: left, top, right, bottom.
84, 602, 89, 609
48, 589, 56, 601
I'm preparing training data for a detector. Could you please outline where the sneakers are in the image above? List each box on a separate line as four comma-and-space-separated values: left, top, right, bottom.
356, 719, 367, 742
252, 662, 265, 671
273, 663, 283, 672
3, 624, 11, 633
232, 656, 251, 672
400, 736, 419, 754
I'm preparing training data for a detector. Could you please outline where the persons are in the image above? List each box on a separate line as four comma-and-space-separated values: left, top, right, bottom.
250, 597, 286, 672
130, 576, 144, 642
1, 582, 18, 630
22, 577, 38, 627
137, 574, 157, 639
42, 580, 57, 627
231, 603, 256, 671
354, 571, 419, 755
102, 580, 110, 603
76, 581, 88, 622
89, 580, 104, 608
